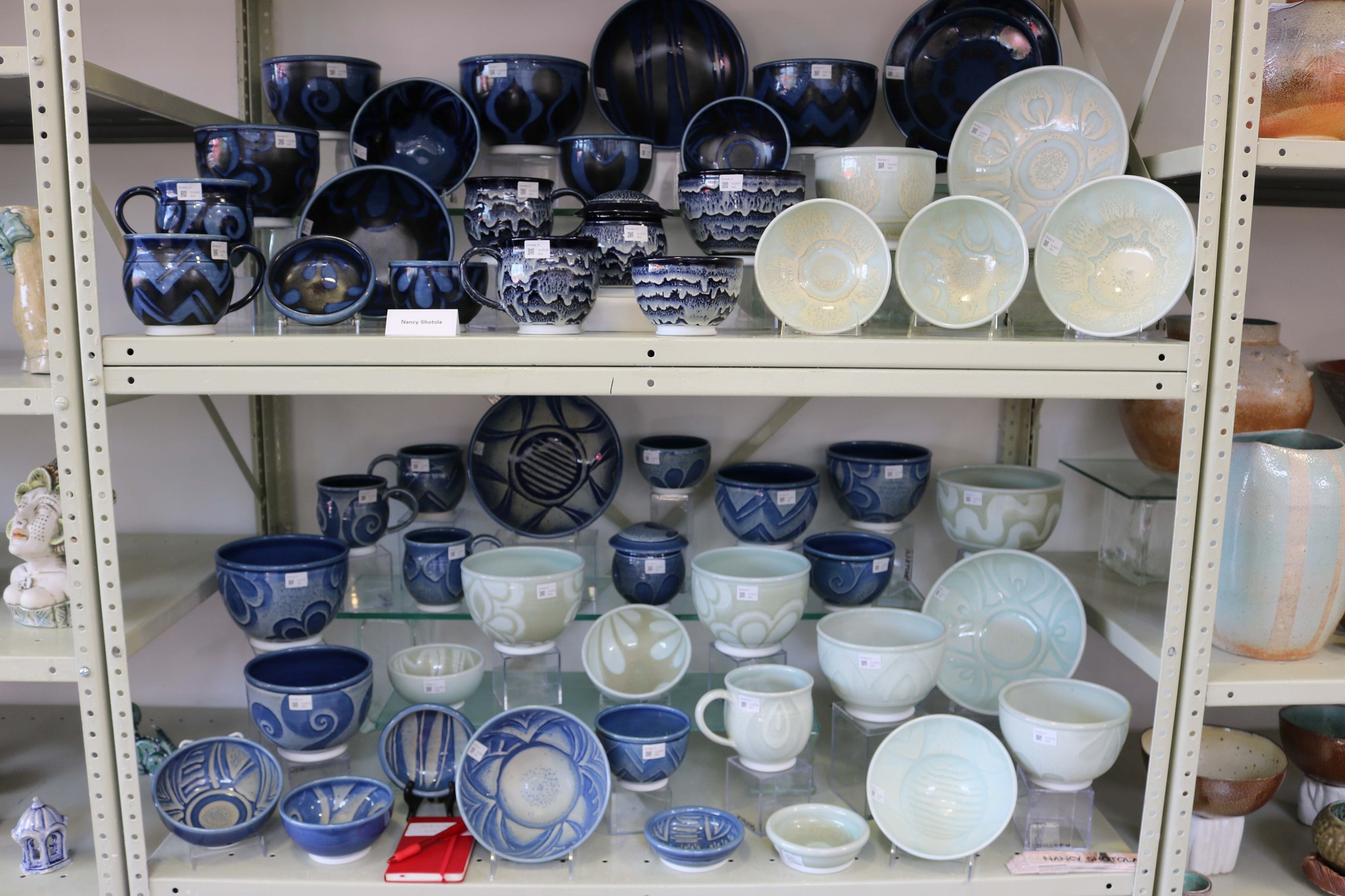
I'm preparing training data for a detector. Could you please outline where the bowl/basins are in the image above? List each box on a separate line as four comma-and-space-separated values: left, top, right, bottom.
143, 435, 1135, 873
1313, 358, 1345, 426
1141, 704, 1345, 815
1182, 868, 1213, 896
192, 53, 938, 342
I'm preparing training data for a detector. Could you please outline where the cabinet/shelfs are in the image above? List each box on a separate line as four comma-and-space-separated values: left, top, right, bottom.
0, 0, 1345, 896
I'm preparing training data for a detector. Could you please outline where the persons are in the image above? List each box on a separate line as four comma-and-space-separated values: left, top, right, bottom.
3, 458, 81, 611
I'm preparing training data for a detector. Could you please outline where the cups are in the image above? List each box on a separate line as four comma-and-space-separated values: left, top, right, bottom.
367, 442, 467, 521
693, 663, 816, 773
119, 230, 266, 337
116, 177, 254, 267
315, 473, 419, 558
458, 236, 600, 335
461, 174, 587, 257
402, 526, 504, 614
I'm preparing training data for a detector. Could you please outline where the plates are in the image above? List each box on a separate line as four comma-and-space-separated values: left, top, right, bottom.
589, 0, 749, 150
883, 0, 1199, 337
753, 197, 892, 335
454, 704, 612, 865
468, 395, 625, 542
921, 548, 1089, 717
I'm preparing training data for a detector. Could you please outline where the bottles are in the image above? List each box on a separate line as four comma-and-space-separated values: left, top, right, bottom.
608, 521, 690, 612
573, 191, 670, 288
1259, 0, 1345, 141
1118, 315, 1318, 478
1214, 427, 1345, 660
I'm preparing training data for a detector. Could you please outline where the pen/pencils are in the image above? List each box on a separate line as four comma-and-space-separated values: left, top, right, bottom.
387, 821, 466, 864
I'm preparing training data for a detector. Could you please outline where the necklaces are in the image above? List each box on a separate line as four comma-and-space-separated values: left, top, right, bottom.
18, 562, 52, 591
